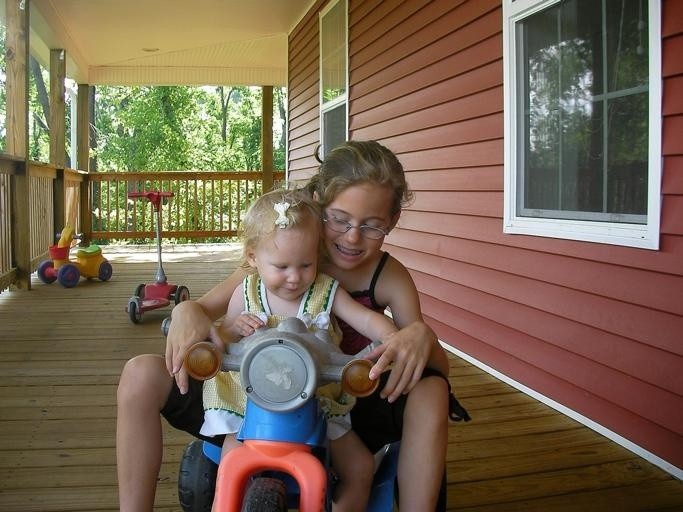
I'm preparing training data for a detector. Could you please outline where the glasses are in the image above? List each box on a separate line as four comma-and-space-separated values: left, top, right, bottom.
322, 210, 395, 240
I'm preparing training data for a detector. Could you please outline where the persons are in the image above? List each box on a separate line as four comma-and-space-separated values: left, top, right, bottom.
198, 183, 399, 512
115, 140, 450, 512
